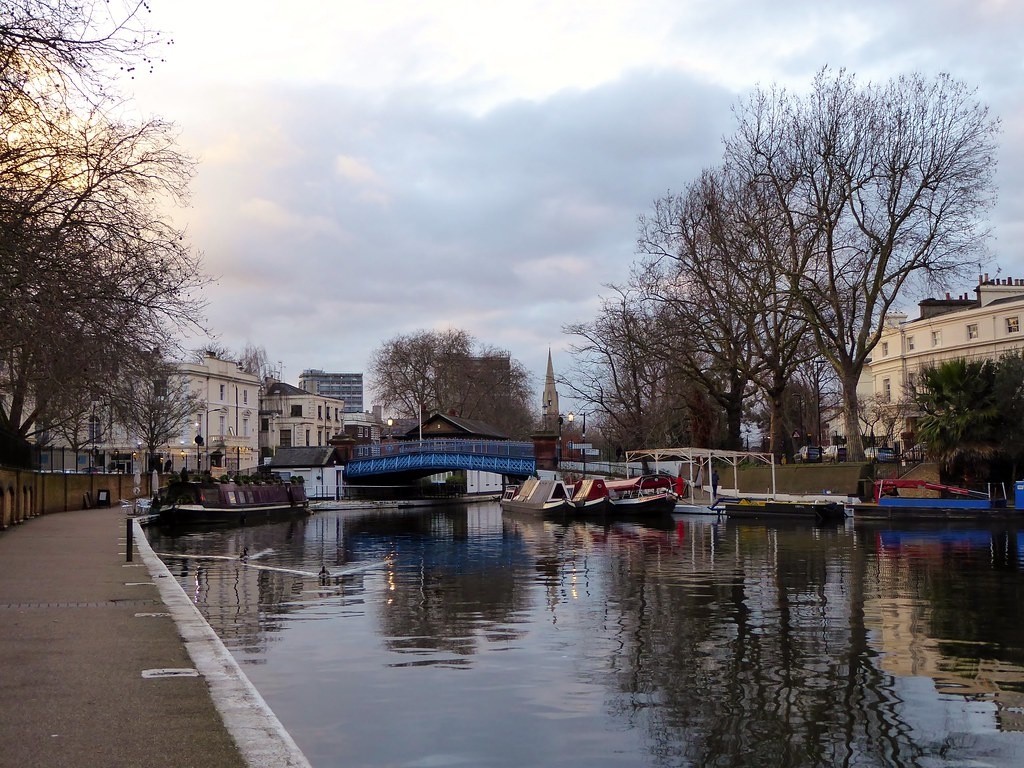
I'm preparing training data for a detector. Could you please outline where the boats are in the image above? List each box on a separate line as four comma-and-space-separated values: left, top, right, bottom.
499, 475, 676, 518
841, 479, 1008, 522
707, 486, 848, 521
159, 479, 314, 527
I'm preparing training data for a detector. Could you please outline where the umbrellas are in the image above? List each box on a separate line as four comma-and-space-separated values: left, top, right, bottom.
152, 469, 159, 499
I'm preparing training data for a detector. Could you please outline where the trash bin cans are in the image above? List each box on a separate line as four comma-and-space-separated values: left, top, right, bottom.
1013, 480, 1024, 510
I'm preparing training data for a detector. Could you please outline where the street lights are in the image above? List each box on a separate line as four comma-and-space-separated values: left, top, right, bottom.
205, 408, 227, 470
387, 416, 393, 443
814, 360, 826, 463
568, 412, 574, 462
579, 411, 586, 478
114, 449, 120, 473
793, 392, 804, 446
180, 448, 188, 471
132, 450, 137, 473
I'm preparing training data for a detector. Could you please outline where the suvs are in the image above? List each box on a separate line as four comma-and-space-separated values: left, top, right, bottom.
905, 442, 928, 462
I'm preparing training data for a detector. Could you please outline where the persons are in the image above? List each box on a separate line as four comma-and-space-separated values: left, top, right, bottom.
92, 461, 94, 467
668, 474, 685, 500
181, 467, 189, 482
712, 470, 719, 499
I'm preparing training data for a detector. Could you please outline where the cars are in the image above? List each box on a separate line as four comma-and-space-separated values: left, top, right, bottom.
794, 446, 837, 463
865, 447, 898, 464
